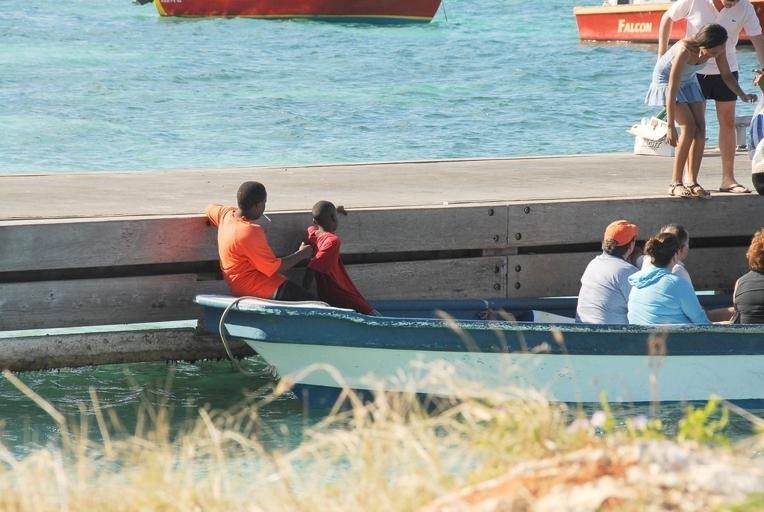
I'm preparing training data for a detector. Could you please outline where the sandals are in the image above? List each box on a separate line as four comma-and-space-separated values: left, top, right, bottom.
666, 183, 710, 198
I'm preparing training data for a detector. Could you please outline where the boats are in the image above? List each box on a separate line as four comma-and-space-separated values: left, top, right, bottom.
573, 0, 764, 44
153, 0, 442, 23
193, 290, 764, 414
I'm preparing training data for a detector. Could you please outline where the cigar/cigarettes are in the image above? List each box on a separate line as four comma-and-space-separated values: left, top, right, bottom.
262, 213, 272, 223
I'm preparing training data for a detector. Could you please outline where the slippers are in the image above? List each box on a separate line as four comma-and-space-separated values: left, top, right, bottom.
719, 185, 750, 193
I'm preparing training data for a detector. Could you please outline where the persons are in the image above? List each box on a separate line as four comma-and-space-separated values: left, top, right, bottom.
304, 200, 382, 313
625, 231, 713, 324
207, 181, 315, 301
727, 226, 764, 324
574, 219, 639, 325
644, 1, 764, 198
634, 224, 695, 292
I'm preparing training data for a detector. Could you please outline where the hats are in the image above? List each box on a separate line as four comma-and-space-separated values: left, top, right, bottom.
602, 220, 638, 246
